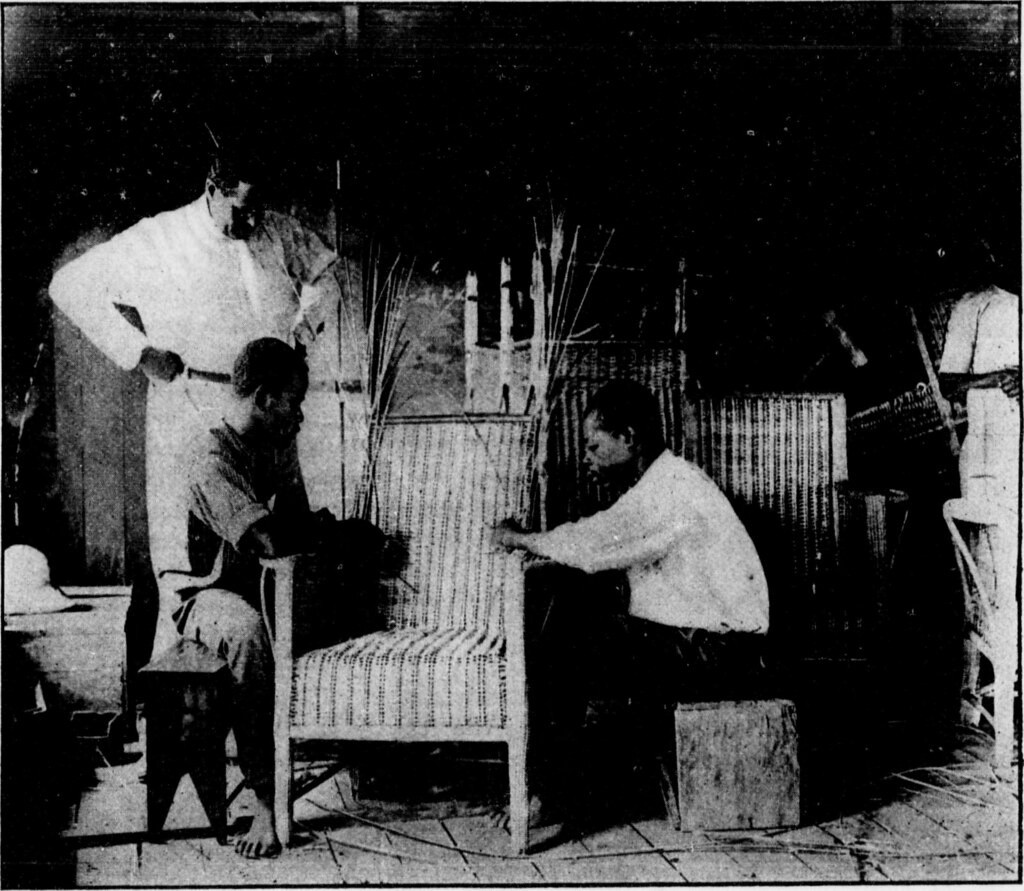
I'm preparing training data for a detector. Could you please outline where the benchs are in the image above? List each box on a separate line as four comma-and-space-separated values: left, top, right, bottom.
136, 637, 246, 847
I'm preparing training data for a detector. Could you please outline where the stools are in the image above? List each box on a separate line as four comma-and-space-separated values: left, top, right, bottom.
673, 696, 801, 833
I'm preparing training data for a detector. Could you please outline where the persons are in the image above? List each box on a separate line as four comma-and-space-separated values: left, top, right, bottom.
157, 332, 336, 861
937, 238, 1021, 729
487, 376, 771, 832
47, 150, 340, 781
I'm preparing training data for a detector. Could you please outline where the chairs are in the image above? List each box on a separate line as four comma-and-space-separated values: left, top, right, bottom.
698, 387, 896, 739
261, 412, 552, 856
